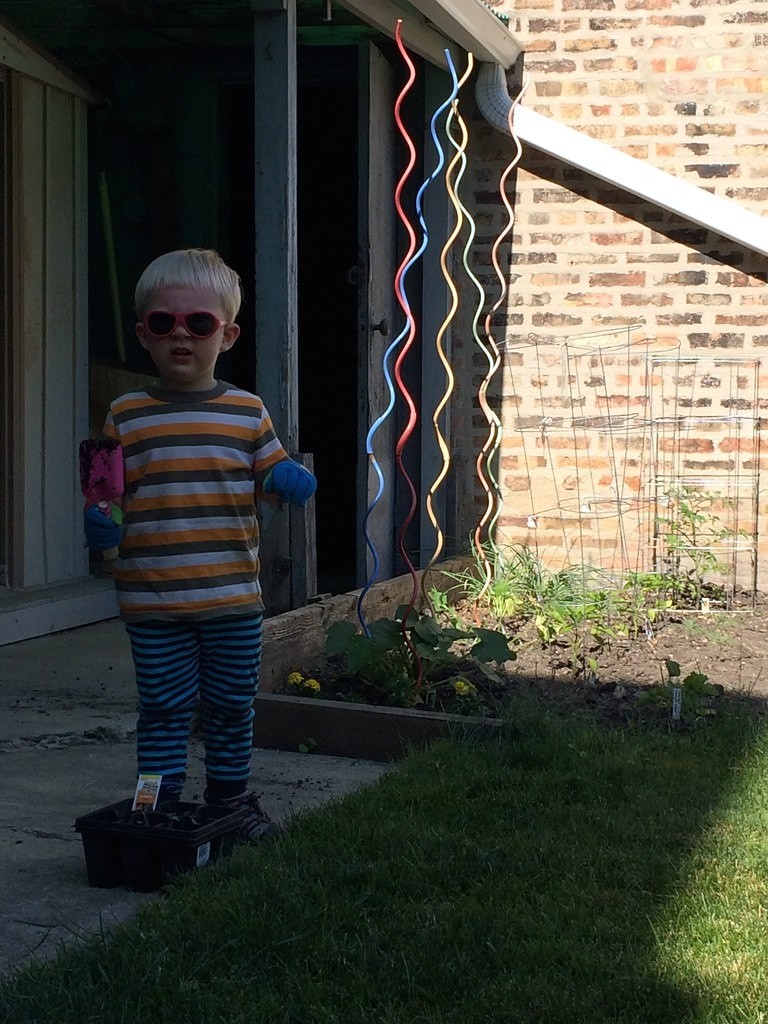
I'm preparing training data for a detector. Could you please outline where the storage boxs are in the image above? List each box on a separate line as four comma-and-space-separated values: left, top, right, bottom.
72, 796, 253, 893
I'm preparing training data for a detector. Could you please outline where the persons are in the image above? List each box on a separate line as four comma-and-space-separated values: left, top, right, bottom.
84, 250, 316, 847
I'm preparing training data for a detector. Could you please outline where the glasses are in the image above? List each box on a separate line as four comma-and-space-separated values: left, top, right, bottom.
143, 309, 225, 338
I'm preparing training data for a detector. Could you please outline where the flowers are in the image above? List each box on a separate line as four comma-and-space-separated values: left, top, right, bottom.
455, 681, 482, 717
289, 672, 321, 698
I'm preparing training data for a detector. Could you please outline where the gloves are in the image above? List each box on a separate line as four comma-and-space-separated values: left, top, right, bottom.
263, 460, 318, 506
83, 503, 123, 551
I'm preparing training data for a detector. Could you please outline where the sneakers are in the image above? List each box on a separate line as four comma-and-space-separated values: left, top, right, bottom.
202, 786, 285, 845
